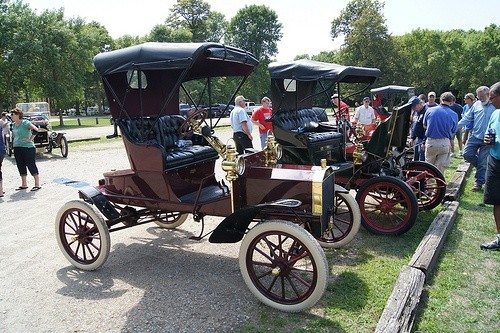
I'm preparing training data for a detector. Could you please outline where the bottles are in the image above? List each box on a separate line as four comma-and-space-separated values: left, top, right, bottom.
175, 139, 192, 148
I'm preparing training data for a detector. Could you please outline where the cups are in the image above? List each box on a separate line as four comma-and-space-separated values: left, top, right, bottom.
485, 132, 495, 145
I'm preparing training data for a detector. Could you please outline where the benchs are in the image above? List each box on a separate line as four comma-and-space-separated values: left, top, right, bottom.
120, 114, 220, 172
275, 107, 343, 148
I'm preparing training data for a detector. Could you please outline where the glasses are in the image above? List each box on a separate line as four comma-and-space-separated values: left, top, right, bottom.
11, 114, 16, 115
365, 101, 369, 103
489, 95, 498, 101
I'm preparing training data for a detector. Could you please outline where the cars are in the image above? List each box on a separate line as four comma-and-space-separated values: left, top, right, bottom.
5, 101, 68, 158
53, 40, 450, 314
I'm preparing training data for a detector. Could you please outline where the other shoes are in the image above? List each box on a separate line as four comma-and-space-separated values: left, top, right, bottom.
459, 152, 463, 155
472, 184, 481, 190
480, 240, 500, 251
451, 154, 456, 157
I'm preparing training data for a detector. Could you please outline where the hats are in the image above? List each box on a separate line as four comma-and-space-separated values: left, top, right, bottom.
363, 97, 369, 101
409, 96, 420, 108
235, 96, 248, 102
428, 91, 436, 97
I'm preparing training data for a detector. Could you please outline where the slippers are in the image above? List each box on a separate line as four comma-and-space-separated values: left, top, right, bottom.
31, 186, 41, 191
16, 187, 28, 190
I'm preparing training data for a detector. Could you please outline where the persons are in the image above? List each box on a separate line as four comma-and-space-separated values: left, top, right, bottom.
251, 97, 272, 150
28, 104, 50, 141
354, 97, 375, 125
10, 108, 41, 191
407, 92, 477, 196
458, 86, 496, 191
331, 94, 351, 133
0, 112, 14, 154
0, 125, 6, 197
230, 96, 254, 154
480, 81, 500, 251
